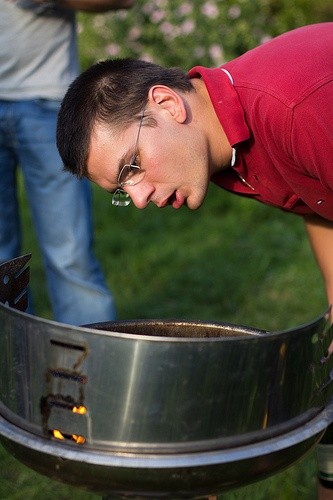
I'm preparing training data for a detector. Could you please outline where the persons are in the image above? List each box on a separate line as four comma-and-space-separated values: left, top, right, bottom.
2, 0, 132, 329
55, 21, 332, 358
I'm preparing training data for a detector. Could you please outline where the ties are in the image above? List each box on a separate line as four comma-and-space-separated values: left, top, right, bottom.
110, 97, 147, 207
